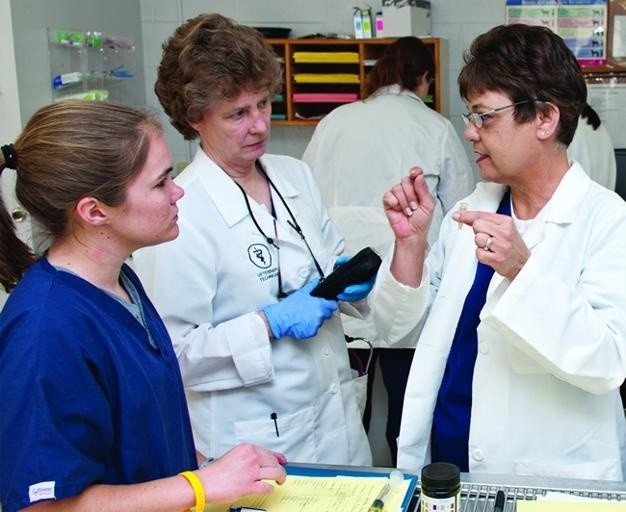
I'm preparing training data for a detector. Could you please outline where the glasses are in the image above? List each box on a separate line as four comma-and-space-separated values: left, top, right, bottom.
462, 99, 544, 129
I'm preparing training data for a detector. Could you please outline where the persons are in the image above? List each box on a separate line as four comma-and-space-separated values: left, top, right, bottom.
376, 24, 626, 484
0, 96, 293, 510
125, 14, 376, 475
297, 30, 479, 470
565, 103, 618, 195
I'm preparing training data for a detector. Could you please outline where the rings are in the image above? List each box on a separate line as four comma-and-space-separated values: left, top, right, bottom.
482, 236, 494, 252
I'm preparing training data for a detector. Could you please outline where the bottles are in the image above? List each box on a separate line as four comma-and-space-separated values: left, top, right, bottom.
362, 10, 384, 39
421, 462, 460, 512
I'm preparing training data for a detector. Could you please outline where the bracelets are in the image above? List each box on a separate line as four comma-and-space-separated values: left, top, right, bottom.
174, 468, 207, 510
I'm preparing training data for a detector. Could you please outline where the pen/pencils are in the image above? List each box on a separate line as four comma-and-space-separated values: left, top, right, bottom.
494, 490, 505, 512
228, 506, 267, 512
271, 413, 279, 437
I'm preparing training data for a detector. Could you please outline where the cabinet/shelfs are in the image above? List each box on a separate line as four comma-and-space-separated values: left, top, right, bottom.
264, 37, 441, 127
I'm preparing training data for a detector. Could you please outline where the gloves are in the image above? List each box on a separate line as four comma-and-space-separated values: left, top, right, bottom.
264, 278, 337, 339
332, 257, 375, 302
